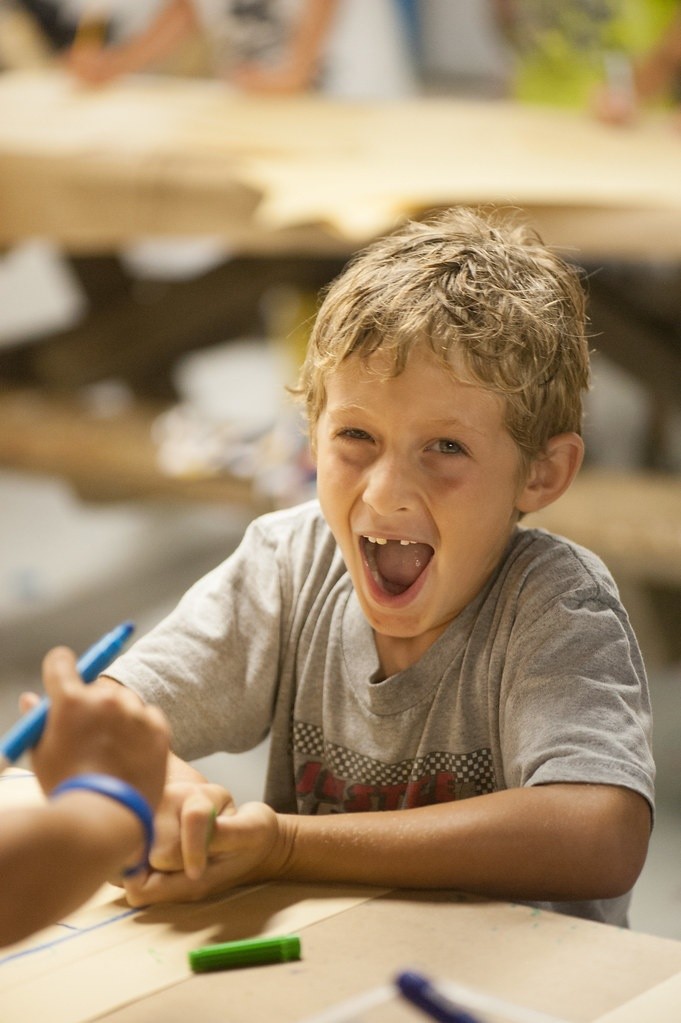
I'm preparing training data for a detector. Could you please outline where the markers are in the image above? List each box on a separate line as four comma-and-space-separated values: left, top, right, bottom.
399, 971, 480, 1023
0, 619, 136, 774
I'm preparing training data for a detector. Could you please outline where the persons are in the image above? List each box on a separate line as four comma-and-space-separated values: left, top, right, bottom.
108, 205, 657, 931
0, 647, 170, 948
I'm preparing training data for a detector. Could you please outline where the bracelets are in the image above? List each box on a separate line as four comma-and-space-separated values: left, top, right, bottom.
52, 774, 155, 877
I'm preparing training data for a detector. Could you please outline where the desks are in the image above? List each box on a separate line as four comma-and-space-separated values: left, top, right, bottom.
0, 764, 681, 1023
